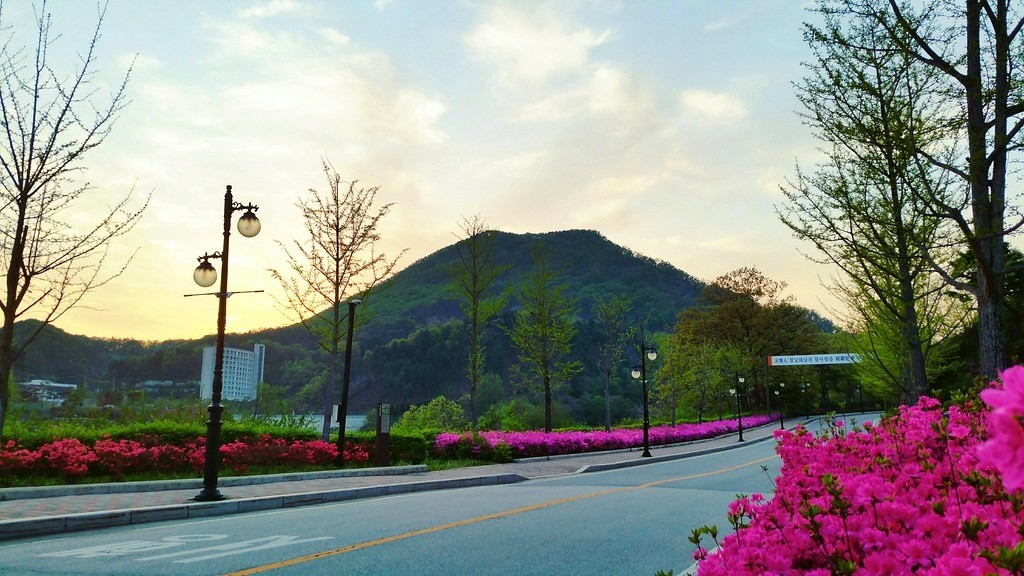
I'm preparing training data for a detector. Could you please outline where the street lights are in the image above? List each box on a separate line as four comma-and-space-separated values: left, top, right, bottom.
195, 184, 262, 498
801, 380, 811, 420
632, 320, 659, 457
775, 378, 785, 430
730, 370, 745, 442
